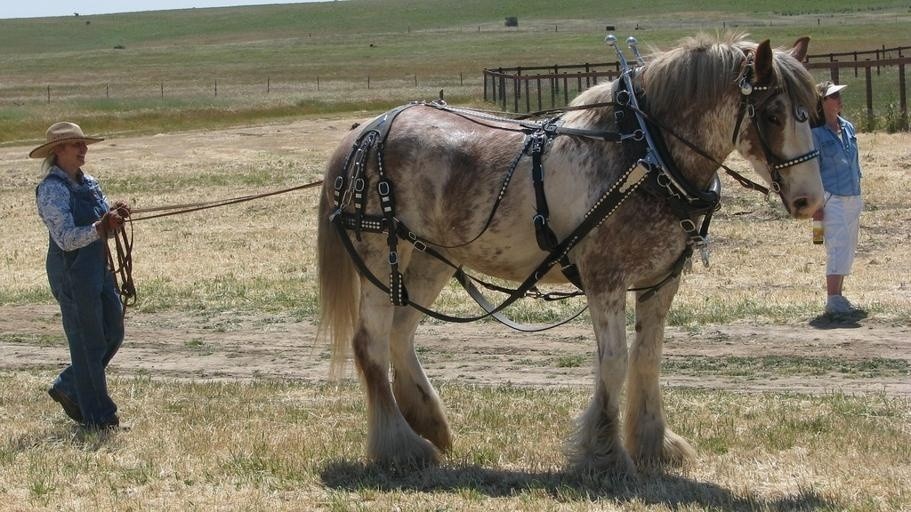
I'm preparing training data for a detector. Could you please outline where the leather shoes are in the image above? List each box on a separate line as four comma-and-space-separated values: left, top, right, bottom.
48, 384, 85, 424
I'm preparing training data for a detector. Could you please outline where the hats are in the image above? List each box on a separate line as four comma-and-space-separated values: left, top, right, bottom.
29, 122, 106, 158
816, 81, 848, 97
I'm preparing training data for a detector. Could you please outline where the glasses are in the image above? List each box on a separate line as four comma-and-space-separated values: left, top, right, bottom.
823, 92, 840, 99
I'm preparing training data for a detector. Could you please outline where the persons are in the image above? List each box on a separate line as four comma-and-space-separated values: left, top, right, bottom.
812, 80, 868, 319
29, 122, 133, 432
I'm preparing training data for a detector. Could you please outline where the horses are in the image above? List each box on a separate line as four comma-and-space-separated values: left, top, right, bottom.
312, 23, 830, 493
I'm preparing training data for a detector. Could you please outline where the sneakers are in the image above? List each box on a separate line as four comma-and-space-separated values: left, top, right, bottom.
825, 296, 859, 313
